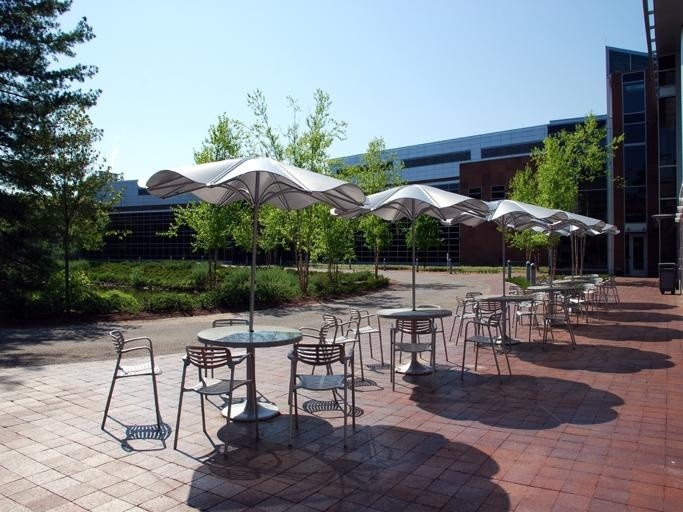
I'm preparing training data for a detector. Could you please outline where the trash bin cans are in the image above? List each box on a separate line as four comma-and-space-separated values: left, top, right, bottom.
658, 263, 678, 295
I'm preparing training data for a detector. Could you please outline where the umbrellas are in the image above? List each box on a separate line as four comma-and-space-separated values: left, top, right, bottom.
436, 198, 569, 336
532, 221, 620, 277
506, 211, 606, 286
135, 155, 368, 407
326, 182, 492, 361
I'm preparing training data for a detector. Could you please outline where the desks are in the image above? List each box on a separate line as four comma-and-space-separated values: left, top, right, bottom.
477, 294, 534, 348
378, 307, 452, 374
197, 325, 303, 422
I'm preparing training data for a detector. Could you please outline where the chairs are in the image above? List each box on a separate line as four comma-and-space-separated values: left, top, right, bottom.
101, 329, 163, 430
388, 317, 436, 395
457, 298, 512, 384
312, 309, 360, 380
449, 292, 484, 345
392, 303, 449, 364
482, 273, 620, 346
349, 306, 384, 368
173, 346, 260, 461
291, 342, 359, 450
210, 318, 249, 378
